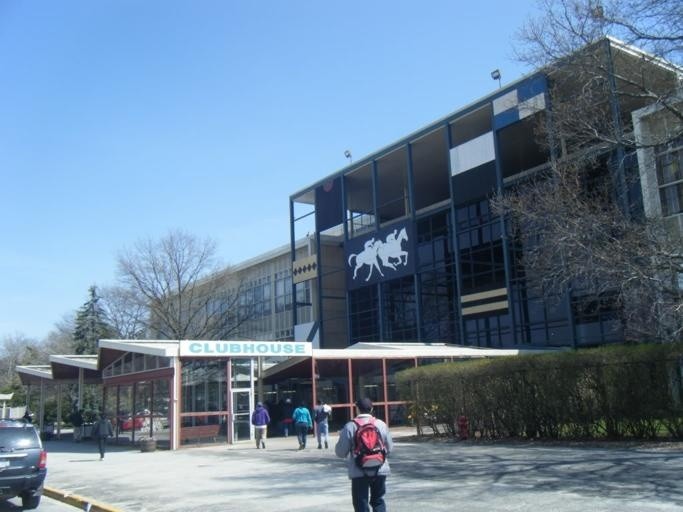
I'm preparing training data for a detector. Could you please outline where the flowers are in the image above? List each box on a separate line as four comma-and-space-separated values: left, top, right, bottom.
140, 418, 163, 439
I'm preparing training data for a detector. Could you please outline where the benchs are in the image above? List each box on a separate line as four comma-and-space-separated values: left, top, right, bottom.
180, 424, 221, 445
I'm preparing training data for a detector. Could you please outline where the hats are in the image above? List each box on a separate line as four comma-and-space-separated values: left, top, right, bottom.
356, 398, 373, 413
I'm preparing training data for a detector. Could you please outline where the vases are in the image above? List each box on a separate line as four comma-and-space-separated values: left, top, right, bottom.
140, 439, 156, 451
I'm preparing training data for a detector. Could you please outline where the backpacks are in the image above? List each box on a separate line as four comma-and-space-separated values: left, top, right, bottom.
349, 417, 389, 470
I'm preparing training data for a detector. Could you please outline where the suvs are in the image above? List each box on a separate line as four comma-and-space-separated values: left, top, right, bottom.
0, 420, 48, 512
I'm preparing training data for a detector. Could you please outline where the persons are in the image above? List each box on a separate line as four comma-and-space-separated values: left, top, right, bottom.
23, 410, 122, 459
335, 398, 393, 512
252, 398, 329, 449
